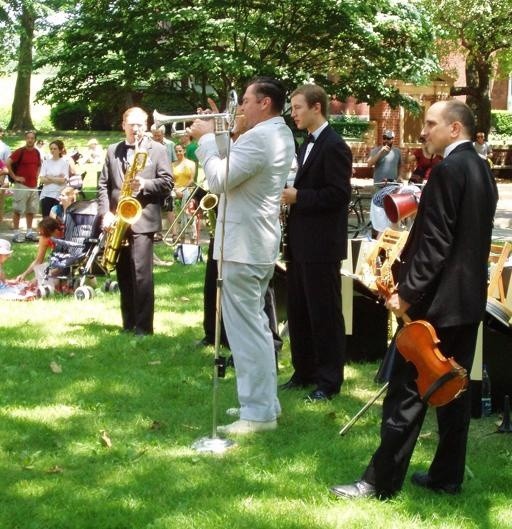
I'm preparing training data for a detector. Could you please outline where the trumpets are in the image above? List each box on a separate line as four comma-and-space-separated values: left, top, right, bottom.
153, 110, 245, 138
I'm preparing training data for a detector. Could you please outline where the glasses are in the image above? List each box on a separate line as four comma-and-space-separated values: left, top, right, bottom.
383, 137, 393, 140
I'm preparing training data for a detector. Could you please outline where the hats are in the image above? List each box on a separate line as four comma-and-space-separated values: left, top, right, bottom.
0, 239, 14, 255
383, 129, 395, 138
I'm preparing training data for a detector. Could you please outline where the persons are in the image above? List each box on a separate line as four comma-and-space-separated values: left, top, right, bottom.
327, 99, 499, 499
187, 81, 296, 438
280, 83, 352, 403
1, 102, 494, 348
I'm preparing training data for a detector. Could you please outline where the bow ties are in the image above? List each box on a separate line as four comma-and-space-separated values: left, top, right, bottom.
303, 132, 315, 144
123, 145, 135, 150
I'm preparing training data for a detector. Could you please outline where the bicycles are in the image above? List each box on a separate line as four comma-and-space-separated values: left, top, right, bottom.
347, 178, 397, 238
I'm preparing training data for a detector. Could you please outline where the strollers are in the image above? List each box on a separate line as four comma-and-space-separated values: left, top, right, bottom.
33, 198, 119, 300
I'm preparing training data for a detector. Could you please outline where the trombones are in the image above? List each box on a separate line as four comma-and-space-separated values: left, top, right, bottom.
163, 178, 210, 247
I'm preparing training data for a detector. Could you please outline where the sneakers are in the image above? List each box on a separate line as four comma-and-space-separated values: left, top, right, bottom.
13, 233, 25, 243
25, 232, 39, 242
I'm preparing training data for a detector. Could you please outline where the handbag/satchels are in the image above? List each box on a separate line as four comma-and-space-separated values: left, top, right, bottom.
174, 243, 202, 265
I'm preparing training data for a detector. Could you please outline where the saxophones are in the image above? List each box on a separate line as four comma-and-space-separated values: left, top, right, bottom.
200, 193, 219, 243
100, 132, 147, 272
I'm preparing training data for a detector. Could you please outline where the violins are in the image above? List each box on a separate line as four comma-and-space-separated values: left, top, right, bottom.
375, 278, 469, 406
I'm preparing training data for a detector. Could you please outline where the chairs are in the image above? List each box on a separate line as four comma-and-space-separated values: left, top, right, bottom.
356, 226, 410, 292
486, 242, 512, 304
